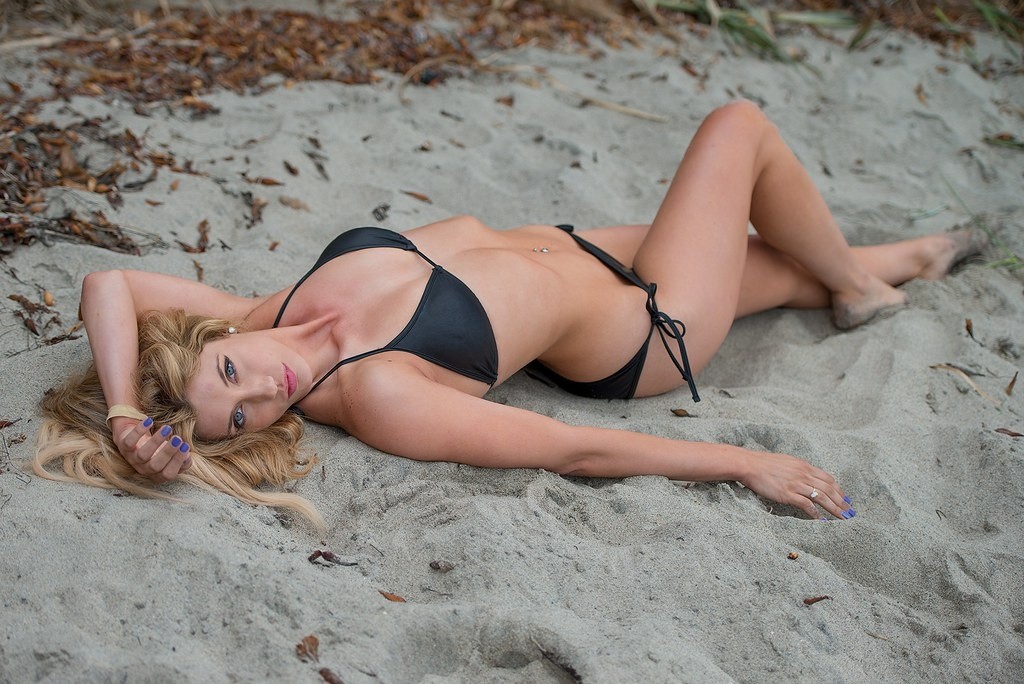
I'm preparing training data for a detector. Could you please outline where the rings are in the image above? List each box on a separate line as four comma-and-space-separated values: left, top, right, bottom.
809, 487, 818, 500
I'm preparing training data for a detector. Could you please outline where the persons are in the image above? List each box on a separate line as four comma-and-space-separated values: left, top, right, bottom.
30, 98, 1003, 521
